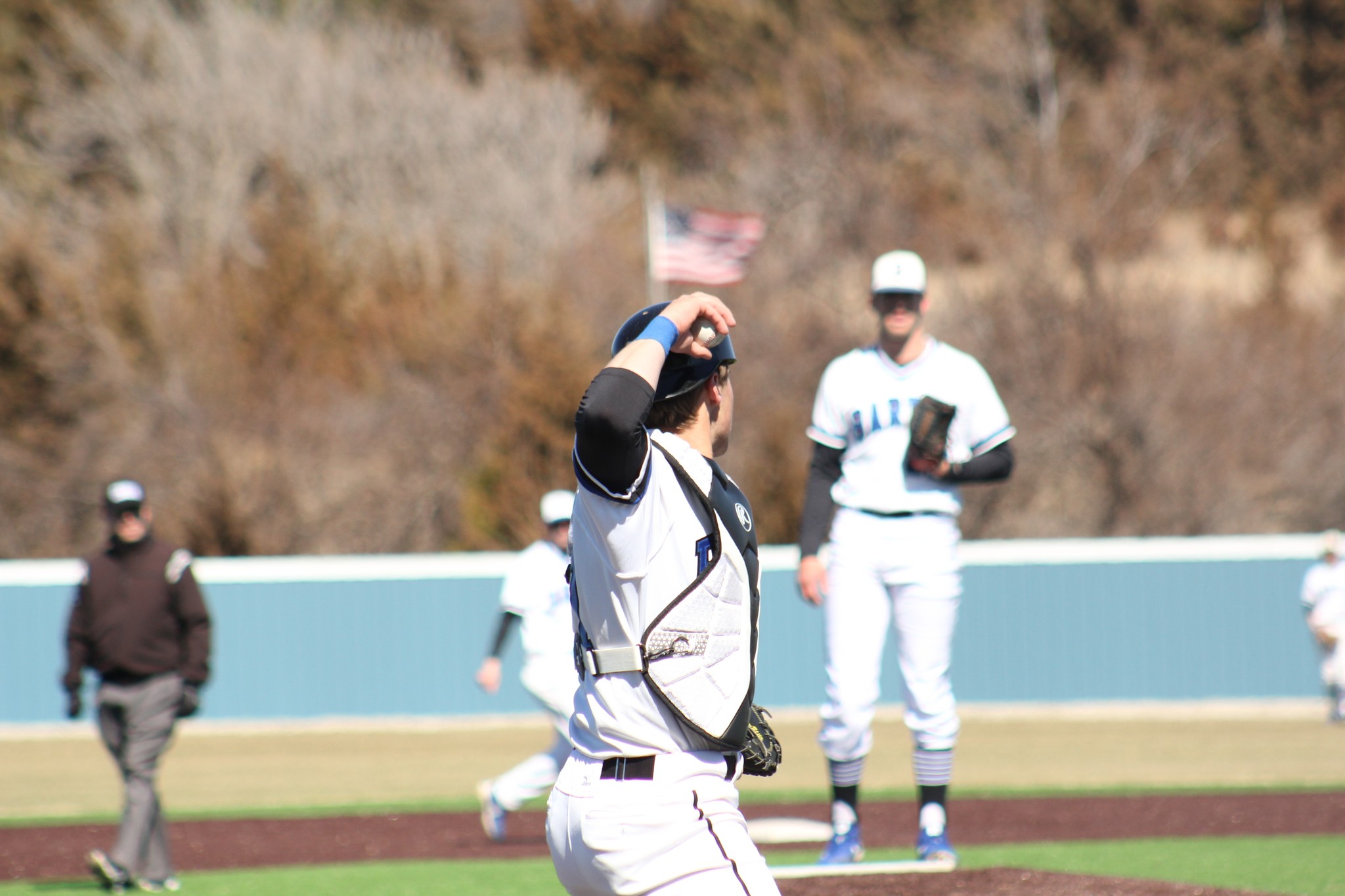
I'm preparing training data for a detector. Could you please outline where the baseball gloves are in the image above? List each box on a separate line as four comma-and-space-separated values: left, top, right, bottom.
903, 396, 956, 478
739, 703, 783, 778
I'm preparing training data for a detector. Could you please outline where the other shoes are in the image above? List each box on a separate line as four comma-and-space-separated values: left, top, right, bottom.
473, 781, 505, 842
86, 849, 135, 891
132, 875, 180, 894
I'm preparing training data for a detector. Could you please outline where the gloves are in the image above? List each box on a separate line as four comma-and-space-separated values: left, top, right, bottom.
173, 684, 198, 717
67, 691, 80, 717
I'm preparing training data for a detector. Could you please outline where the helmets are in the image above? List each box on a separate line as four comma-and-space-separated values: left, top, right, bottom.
610, 302, 737, 403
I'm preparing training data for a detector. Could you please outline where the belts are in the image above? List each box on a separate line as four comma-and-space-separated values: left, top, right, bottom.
859, 508, 943, 519
600, 754, 744, 781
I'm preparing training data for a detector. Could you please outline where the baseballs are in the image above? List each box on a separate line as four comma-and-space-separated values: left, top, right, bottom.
689, 316, 727, 348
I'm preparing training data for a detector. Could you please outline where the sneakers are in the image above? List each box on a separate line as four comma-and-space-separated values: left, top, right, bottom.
912, 826, 958, 864
814, 824, 866, 864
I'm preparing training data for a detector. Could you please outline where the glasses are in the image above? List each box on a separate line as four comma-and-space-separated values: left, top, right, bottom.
108, 503, 142, 518
870, 290, 923, 316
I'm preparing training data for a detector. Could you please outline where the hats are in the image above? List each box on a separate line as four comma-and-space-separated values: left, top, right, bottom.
105, 480, 144, 505
539, 489, 576, 525
1315, 527, 1345, 557
869, 249, 928, 294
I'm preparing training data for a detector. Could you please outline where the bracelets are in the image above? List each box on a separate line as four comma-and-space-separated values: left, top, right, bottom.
632, 316, 679, 357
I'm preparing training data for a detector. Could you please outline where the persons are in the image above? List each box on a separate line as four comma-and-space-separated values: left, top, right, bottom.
797, 250, 1016, 871
478, 488, 582, 842
546, 289, 781, 896
1301, 528, 1345, 722
63, 480, 211, 893
